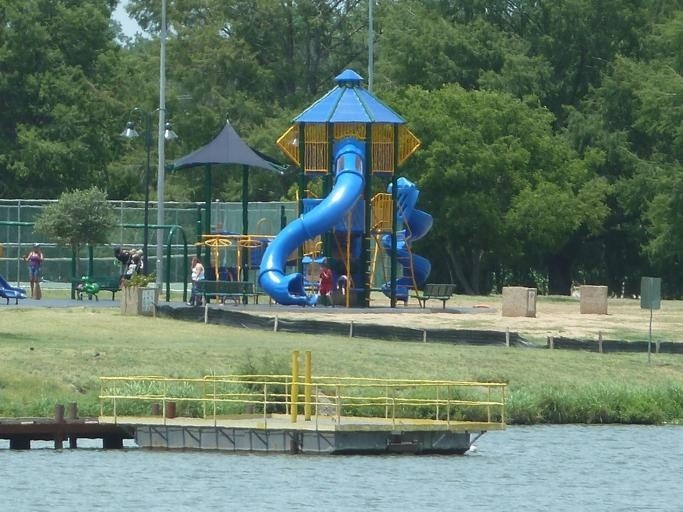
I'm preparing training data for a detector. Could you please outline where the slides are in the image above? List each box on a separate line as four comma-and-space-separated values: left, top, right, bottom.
258, 145, 367, 305
0, 274, 26, 299
382, 208, 433, 301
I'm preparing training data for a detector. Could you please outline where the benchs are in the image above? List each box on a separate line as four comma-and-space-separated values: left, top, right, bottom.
197, 279, 260, 306
411, 283, 456, 309
72, 275, 122, 301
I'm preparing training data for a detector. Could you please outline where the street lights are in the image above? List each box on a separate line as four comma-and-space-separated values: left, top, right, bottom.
117, 105, 180, 281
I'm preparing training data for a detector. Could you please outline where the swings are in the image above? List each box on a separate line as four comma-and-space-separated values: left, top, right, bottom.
120, 227, 156, 282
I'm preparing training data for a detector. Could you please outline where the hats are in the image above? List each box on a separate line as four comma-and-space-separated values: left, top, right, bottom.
33, 242, 39, 247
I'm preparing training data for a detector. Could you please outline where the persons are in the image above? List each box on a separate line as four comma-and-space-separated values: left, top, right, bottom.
113, 245, 143, 265
311, 263, 336, 308
22, 243, 43, 298
186, 256, 206, 307
117, 255, 144, 290
